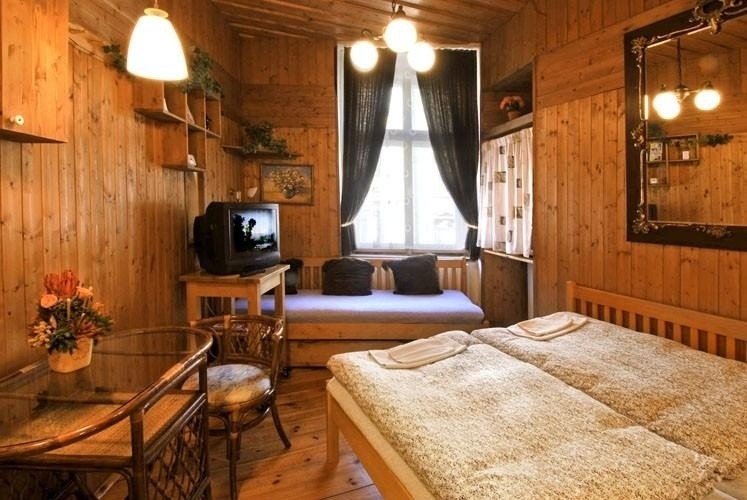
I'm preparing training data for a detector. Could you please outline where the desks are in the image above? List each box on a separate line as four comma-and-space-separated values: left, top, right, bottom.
175, 262, 296, 385
1, 324, 219, 500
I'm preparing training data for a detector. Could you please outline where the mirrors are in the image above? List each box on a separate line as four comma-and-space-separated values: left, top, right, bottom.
616, 0, 747, 254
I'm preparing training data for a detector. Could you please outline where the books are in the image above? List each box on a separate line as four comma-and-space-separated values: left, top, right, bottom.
649, 176, 665, 185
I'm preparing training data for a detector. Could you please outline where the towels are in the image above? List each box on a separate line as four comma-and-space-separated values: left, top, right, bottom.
364, 334, 466, 369
504, 308, 590, 342
388, 338, 452, 363
515, 316, 575, 336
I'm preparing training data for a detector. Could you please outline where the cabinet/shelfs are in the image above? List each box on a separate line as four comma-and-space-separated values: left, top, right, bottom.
128, 67, 223, 172
1, 0, 74, 148
645, 127, 703, 191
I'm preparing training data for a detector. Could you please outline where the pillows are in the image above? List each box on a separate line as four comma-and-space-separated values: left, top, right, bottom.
319, 257, 376, 297
382, 251, 441, 294
257, 259, 306, 297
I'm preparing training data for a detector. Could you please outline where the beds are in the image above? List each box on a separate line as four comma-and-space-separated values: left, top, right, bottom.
314, 279, 747, 500
220, 255, 484, 371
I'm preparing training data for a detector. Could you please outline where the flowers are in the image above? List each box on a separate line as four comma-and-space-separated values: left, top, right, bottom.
23, 263, 116, 353
497, 92, 524, 108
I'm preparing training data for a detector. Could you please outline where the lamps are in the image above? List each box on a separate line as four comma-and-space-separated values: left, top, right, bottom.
349, 0, 436, 81
650, 37, 725, 121
125, 1, 188, 85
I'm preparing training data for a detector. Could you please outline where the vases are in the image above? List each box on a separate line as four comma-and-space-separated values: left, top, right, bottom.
504, 108, 520, 121
47, 339, 94, 373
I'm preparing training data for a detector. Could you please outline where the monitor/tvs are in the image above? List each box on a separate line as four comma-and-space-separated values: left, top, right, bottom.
193, 201, 281, 276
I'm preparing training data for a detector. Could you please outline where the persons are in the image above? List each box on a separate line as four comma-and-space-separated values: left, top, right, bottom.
651, 144, 662, 161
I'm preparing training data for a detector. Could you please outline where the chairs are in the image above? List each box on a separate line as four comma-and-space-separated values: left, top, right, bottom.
182, 312, 294, 500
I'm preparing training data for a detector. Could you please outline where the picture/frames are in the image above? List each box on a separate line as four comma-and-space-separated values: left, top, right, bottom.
256, 160, 315, 207
